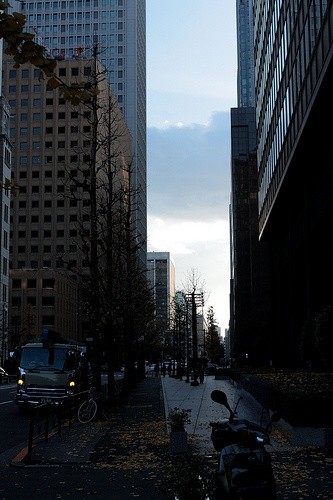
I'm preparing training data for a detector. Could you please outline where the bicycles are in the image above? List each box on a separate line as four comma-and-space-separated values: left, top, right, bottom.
78, 386, 103, 424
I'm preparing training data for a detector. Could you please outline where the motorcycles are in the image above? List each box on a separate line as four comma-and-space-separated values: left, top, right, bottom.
208, 390, 285, 500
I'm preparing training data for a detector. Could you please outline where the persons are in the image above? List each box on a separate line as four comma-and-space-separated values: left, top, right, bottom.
162, 363, 166, 377
167, 364, 172, 377
146, 362, 150, 374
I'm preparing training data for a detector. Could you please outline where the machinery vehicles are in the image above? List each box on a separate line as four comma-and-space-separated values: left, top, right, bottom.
15, 325, 92, 416
